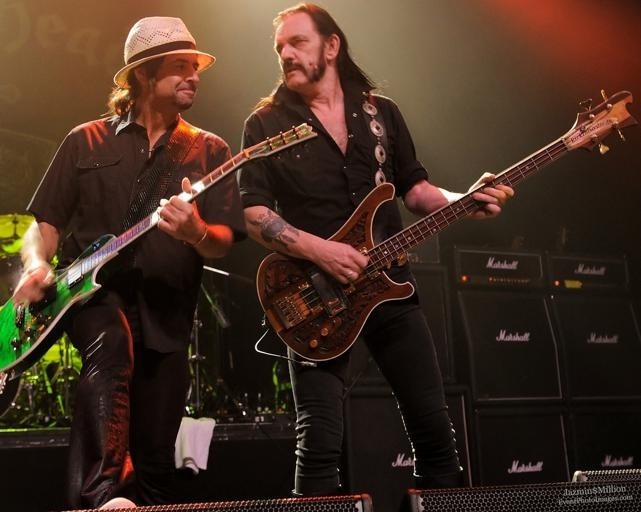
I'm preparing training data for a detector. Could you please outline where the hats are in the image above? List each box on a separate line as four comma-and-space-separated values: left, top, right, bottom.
112, 16, 217, 90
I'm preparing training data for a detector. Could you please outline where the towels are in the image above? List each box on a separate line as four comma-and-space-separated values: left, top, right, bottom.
174, 415, 216, 474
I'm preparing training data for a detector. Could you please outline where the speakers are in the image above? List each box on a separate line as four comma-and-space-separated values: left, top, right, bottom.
547, 254, 641, 400
471, 407, 568, 485
569, 405, 639, 478
344, 385, 474, 509
450, 240, 562, 403
341, 230, 453, 385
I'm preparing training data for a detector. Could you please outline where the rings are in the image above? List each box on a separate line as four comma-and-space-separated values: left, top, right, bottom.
346, 270, 353, 278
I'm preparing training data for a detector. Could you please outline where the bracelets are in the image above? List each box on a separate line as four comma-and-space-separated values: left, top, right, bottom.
182, 223, 209, 248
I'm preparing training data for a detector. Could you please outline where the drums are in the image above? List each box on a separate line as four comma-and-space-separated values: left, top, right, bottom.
0, 367, 38, 426
39, 361, 81, 422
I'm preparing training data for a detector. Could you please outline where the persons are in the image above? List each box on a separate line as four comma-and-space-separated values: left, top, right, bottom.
240, 4, 516, 496
13, 16, 246, 509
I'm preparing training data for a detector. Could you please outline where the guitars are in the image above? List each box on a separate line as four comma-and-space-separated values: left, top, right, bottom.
255, 88, 638, 363
0, 121, 318, 373
